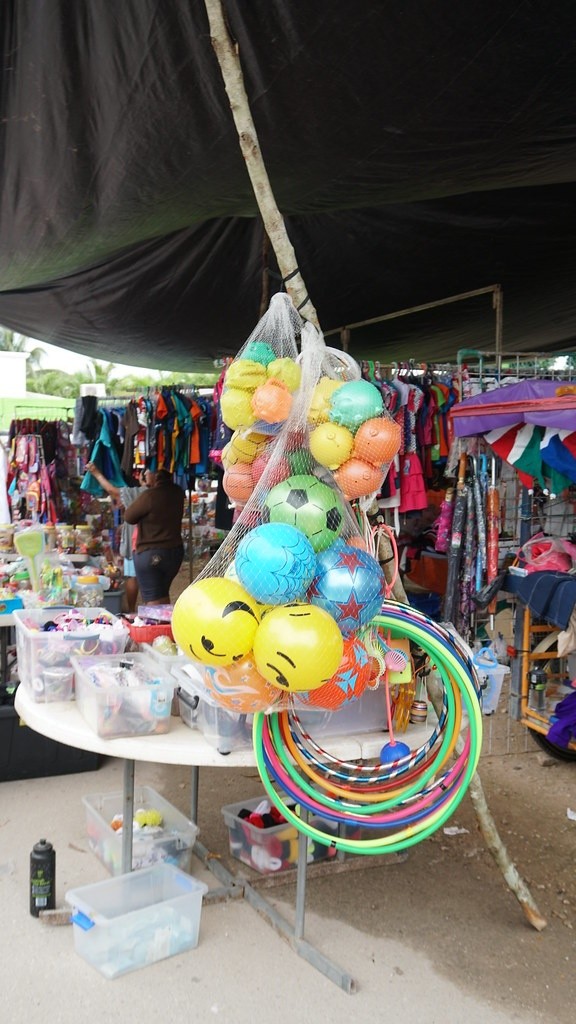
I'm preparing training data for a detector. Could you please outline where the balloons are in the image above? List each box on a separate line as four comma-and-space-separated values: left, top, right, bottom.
166, 294, 403, 715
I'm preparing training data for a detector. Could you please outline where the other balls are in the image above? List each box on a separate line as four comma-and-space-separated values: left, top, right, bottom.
172, 340, 402, 716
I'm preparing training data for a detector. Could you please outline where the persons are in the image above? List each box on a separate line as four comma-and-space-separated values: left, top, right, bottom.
82, 458, 186, 606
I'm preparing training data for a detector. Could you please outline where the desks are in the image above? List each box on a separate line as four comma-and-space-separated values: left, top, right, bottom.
12, 680, 470, 997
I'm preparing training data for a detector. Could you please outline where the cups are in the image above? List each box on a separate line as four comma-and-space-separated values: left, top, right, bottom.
43, 667, 74, 712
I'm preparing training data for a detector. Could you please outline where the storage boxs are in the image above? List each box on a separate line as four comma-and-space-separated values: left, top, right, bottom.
460, 658, 511, 716
13, 606, 131, 702
115, 606, 418, 753
101, 587, 126, 616
65, 862, 210, 981
81, 784, 200, 879
68, 652, 180, 740
220, 781, 361, 874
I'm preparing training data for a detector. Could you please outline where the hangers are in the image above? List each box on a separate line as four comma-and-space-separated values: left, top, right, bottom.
354, 358, 538, 392
95, 382, 207, 414
12, 416, 75, 427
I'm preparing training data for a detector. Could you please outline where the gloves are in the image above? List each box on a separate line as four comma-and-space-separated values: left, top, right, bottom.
229, 796, 362, 875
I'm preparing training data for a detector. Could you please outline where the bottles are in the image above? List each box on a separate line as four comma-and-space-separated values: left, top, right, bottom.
0, 523, 92, 555
30, 840, 55, 917
528, 664, 546, 720
502, 553, 516, 575
73, 575, 102, 608
